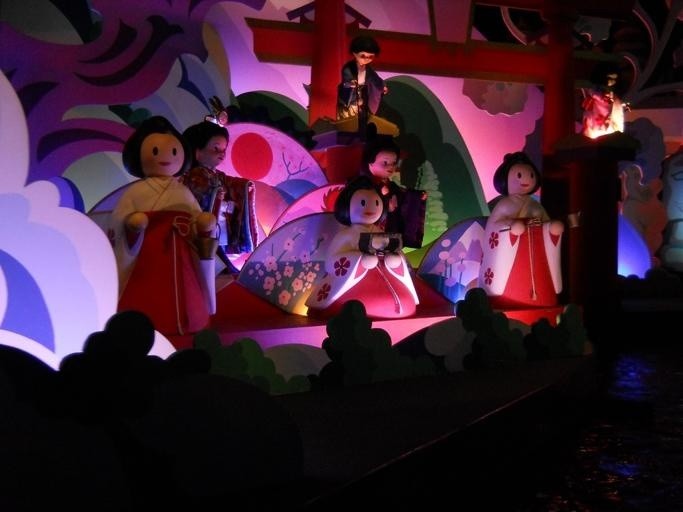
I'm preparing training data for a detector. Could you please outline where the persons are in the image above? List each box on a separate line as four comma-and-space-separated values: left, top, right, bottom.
581, 60, 632, 139
111, 115, 220, 337
336, 34, 389, 118
305, 175, 420, 324
362, 133, 428, 250
180, 109, 259, 253
478, 151, 565, 327
